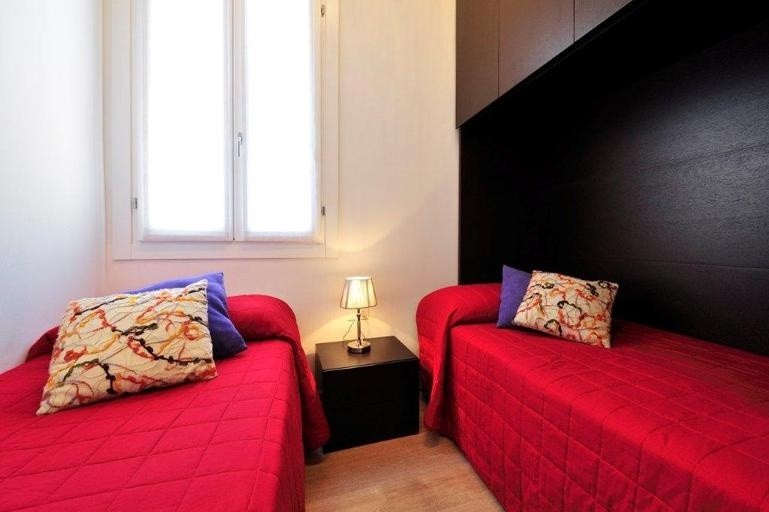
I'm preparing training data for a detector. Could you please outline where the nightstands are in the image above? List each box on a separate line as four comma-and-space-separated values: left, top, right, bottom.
305, 336, 427, 454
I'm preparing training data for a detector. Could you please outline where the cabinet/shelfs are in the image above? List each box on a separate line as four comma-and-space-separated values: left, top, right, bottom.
453, 0, 633, 129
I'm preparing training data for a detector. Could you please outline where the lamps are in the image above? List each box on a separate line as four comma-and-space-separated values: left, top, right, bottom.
338, 274, 381, 353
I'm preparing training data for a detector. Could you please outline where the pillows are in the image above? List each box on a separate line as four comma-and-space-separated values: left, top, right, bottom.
496, 260, 534, 332
509, 266, 620, 350
34, 277, 219, 420
120, 271, 247, 364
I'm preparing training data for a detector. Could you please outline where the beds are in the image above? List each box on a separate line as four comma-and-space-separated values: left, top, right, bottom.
0, 293, 331, 512
414, 281, 768, 511
